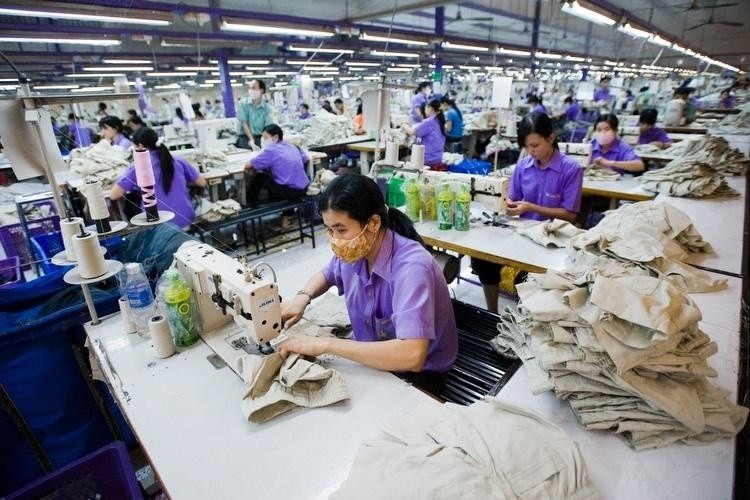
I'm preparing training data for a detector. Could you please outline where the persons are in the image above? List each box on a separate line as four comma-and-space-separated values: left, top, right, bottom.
278, 172, 459, 397
522, 75, 734, 177
470, 111, 584, 314
399, 81, 466, 166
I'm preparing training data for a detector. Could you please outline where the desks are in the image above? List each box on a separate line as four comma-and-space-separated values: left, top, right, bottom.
0, 99, 750, 500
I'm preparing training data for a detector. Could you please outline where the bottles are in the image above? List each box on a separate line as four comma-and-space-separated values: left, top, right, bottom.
455, 184, 471, 231
419, 178, 437, 220
124, 262, 157, 339
405, 178, 420, 222
165, 270, 198, 346
439, 177, 453, 230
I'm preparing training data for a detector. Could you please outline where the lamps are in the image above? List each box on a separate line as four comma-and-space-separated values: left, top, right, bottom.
0, 0, 750, 81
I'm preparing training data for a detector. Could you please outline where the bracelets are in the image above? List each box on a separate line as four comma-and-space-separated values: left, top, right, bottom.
296, 290, 312, 305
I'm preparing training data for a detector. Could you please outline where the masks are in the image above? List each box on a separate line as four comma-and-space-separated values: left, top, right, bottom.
330, 217, 379, 262
596, 130, 615, 145
261, 139, 272, 148
249, 89, 260, 98
